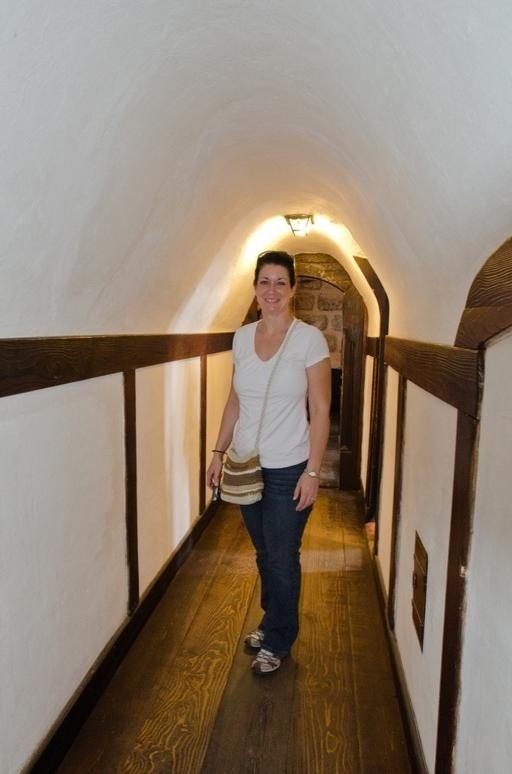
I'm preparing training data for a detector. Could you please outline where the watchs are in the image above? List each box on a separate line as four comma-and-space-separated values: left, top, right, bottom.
304, 470, 319, 478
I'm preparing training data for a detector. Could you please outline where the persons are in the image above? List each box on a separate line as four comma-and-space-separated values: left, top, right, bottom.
204, 251, 332, 676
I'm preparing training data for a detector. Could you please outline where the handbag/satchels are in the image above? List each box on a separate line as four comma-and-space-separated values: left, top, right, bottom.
220, 450, 264, 506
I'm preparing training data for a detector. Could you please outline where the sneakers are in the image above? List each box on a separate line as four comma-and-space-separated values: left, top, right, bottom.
251, 649, 282, 673
244, 629, 264, 648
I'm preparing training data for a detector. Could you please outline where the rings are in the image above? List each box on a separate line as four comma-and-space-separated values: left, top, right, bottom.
310, 497, 317, 504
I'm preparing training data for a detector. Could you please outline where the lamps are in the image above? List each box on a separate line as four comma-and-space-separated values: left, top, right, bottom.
283, 213, 316, 239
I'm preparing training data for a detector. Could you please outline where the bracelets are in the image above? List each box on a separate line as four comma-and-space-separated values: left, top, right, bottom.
211, 450, 226, 455
